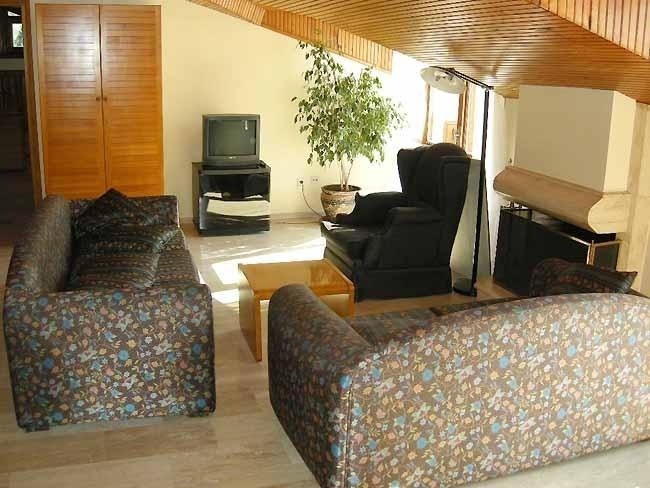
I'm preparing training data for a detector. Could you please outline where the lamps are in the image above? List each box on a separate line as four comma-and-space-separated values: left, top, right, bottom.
420, 64, 495, 298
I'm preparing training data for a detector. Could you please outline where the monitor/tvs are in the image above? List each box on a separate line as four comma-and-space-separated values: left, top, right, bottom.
202, 113, 260, 170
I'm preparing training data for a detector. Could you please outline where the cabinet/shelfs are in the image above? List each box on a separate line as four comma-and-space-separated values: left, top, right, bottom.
189, 158, 273, 237
35, 2, 165, 203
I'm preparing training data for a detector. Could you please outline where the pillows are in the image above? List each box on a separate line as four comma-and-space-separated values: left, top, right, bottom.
529, 258, 640, 302
63, 190, 178, 295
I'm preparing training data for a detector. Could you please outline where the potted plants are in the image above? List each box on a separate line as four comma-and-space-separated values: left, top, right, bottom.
288, 30, 411, 221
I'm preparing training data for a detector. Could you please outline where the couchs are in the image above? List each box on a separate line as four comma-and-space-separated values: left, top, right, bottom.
2, 190, 224, 431
259, 258, 649, 487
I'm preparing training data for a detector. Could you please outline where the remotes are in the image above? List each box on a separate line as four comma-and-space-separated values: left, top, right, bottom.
429, 307, 441, 316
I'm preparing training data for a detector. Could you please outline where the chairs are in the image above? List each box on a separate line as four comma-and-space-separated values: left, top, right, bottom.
321, 139, 472, 303
